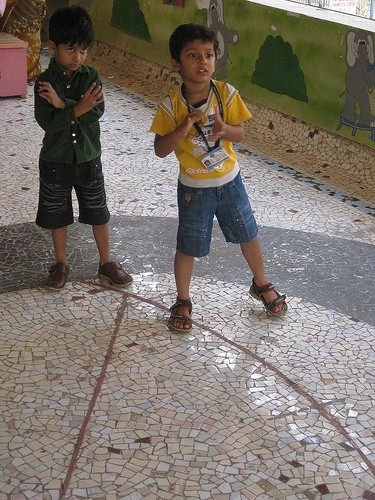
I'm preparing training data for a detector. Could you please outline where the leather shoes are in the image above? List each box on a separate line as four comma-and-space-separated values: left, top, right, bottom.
98, 261, 132, 286
47, 264, 68, 291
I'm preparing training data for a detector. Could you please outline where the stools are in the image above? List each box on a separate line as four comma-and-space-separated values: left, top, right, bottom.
0, 32, 29, 98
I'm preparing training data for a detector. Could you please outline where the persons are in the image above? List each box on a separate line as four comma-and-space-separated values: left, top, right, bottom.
149, 23, 289, 332
32, 4, 134, 287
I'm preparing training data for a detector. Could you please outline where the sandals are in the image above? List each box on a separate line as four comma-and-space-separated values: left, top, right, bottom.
251, 278, 289, 317
168, 297, 194, 333
